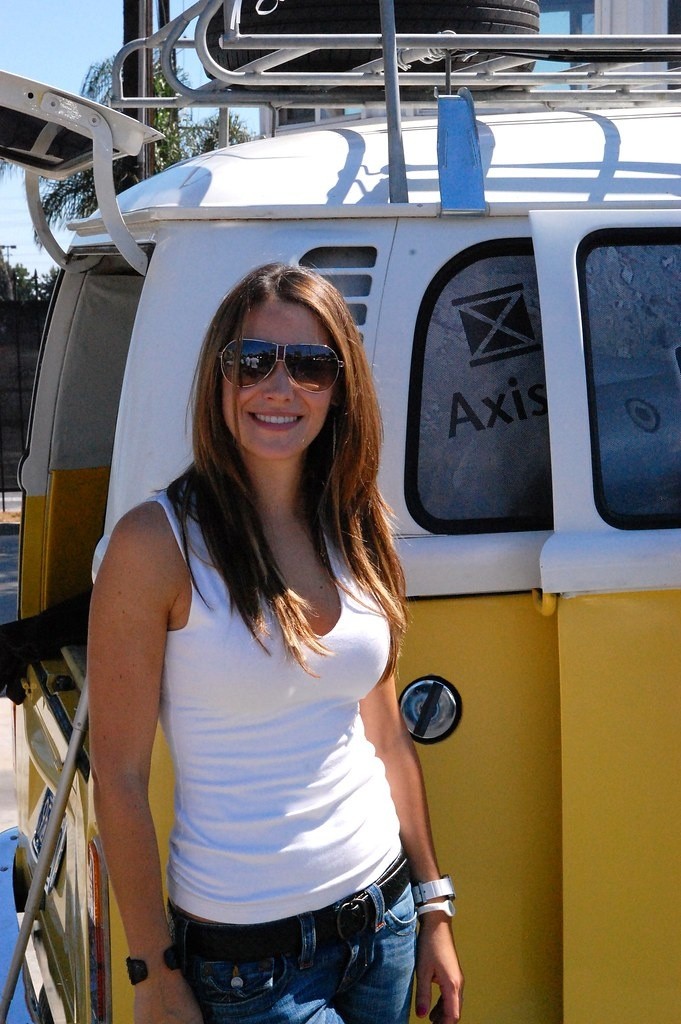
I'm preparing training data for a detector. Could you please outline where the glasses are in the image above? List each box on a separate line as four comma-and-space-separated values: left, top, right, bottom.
218, 338, 345, 394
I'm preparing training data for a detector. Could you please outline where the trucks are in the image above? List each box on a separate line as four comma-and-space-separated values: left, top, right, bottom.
0, 0, 681, 1024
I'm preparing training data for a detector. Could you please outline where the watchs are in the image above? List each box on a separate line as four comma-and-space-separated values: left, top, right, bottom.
412, 873, 456, 917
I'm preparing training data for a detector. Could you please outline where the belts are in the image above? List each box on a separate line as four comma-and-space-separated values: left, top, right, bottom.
168, 857, 410, 963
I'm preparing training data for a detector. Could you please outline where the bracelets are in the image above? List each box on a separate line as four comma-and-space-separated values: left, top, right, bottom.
126, 945, 182, 986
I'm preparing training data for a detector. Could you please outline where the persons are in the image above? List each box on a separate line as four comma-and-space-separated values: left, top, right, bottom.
86, 265, 464, 1024
239, 350, 269, 377
